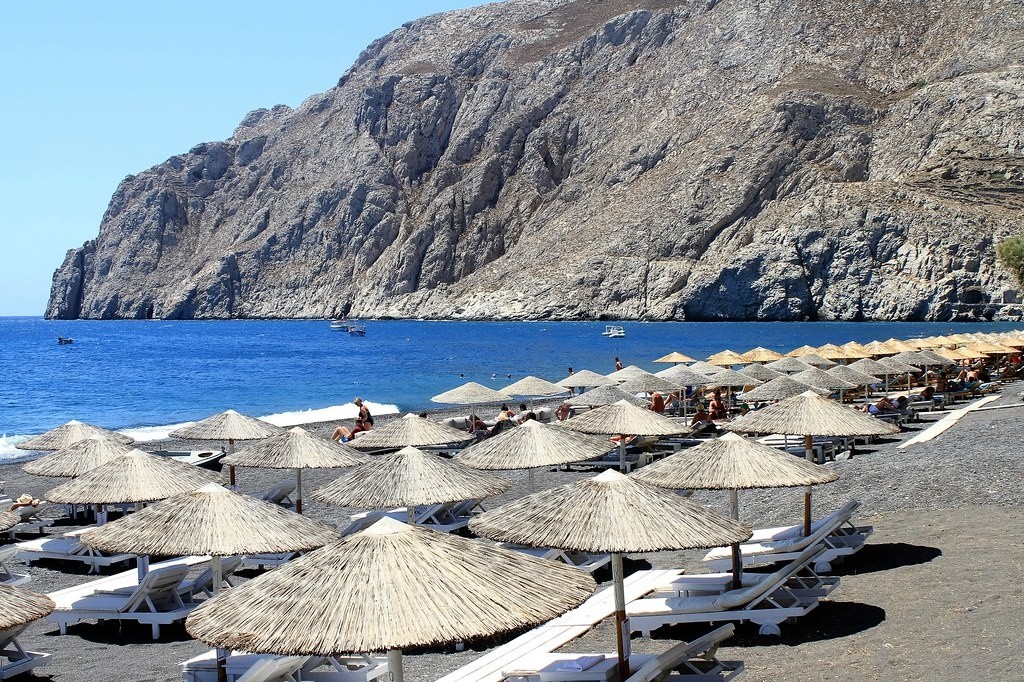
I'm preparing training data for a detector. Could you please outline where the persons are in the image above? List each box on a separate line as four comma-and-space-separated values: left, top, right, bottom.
9, 493, 40, 511
330, 346, 1024, 480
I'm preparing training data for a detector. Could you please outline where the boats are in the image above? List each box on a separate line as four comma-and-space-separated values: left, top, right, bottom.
602, 325, 625, 338
348, 321, 366, 336
57, 336, 72, 345
330, 313, 348, 331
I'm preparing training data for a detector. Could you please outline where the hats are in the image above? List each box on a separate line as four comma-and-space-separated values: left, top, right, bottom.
696, 404, 704, 410
354, 417, 363, 420
354, 398, 360, 403
742, 403, 748, 409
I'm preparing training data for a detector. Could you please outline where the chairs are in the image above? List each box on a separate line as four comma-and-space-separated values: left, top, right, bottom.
0, 359, 1024, 682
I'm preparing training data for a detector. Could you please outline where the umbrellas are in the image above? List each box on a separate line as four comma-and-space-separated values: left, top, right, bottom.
467, 468, 753, 682
498, 330, 1024, 475
722, 391, 901, 535
0, 409, 615, 626
628, 430, 840, 590
185, 516, 598, 682
430, 381, 514, 432
